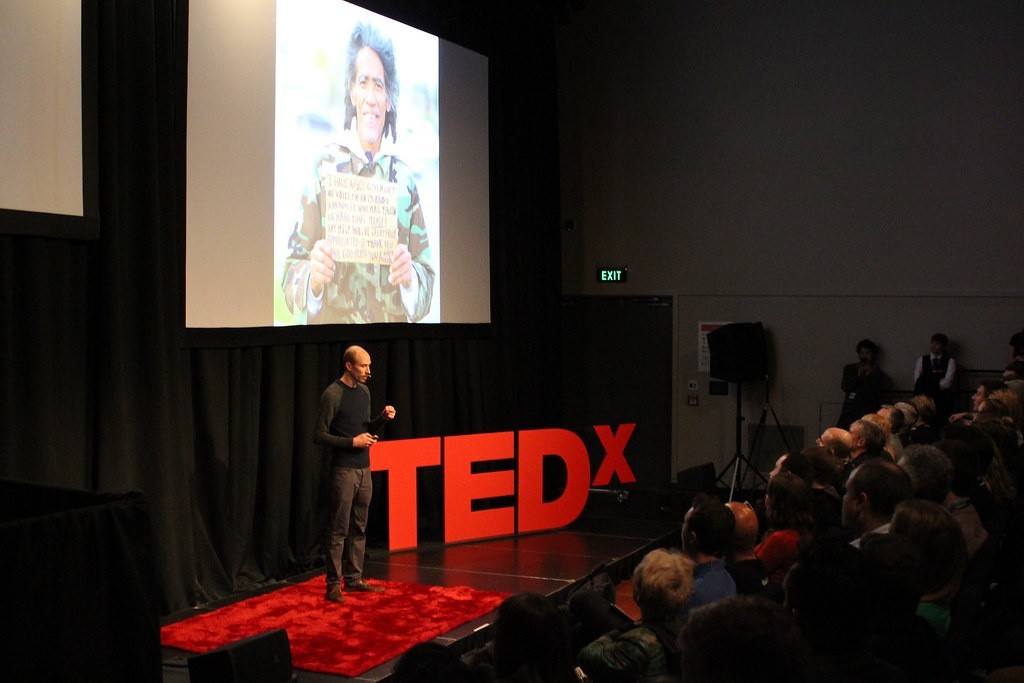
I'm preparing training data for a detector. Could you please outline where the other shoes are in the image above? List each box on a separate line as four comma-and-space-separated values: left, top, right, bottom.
327, 582, 344, 603
344, 580, 377, 593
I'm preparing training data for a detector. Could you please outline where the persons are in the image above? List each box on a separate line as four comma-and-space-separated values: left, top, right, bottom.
280, 21, 437, 325
315, 346, 396, 602
911, 332, 957, 416
835, 339, 895, 431
1000, 331, 1024, 379
386, 377, 1024, 683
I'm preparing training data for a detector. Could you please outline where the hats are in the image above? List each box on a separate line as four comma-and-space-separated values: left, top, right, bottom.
857, 340, 880, 352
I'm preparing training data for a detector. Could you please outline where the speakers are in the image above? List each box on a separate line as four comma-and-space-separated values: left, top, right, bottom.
707, 321, 770, 383
187, 629, 292, 683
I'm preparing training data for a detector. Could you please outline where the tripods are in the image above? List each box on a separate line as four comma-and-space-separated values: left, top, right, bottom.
714, 378, 793, 503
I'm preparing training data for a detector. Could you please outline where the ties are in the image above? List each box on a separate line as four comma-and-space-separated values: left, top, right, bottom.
932, 358, 939, 370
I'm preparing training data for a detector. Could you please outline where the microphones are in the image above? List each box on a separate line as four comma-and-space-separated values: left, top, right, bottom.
368, 375, 371, 377
